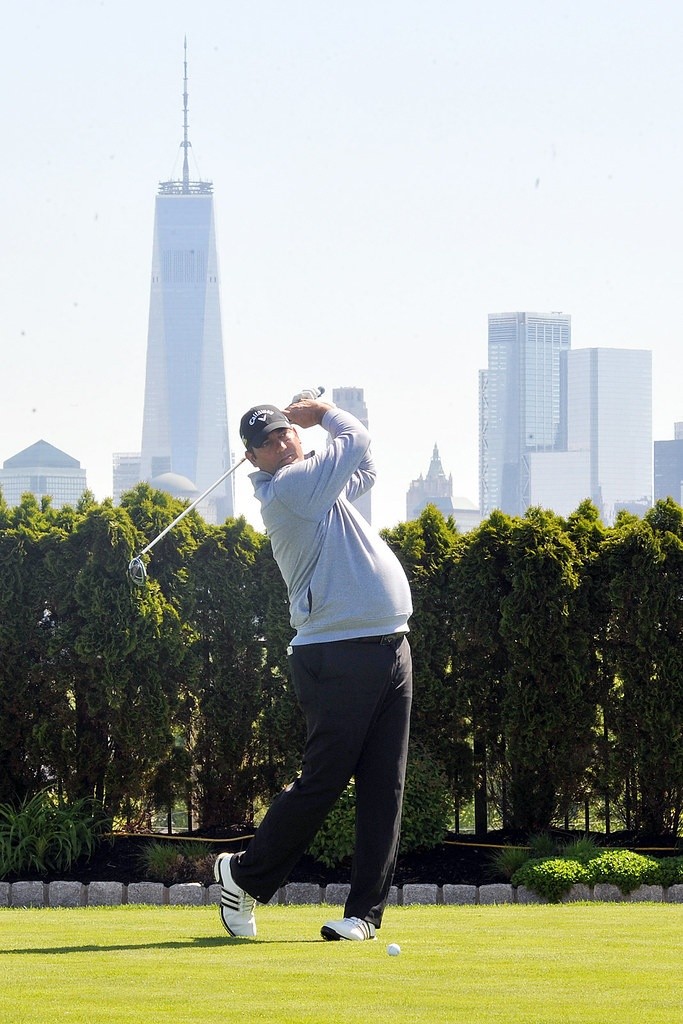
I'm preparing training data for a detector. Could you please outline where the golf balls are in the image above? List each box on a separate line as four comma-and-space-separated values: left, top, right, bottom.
388, 942, 401, 957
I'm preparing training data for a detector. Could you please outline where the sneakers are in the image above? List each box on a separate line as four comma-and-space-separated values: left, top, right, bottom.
213, 852, 257, 939
320, 916, 377, 943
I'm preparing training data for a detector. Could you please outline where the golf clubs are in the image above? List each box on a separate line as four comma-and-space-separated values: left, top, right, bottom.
123, 383, 329, 582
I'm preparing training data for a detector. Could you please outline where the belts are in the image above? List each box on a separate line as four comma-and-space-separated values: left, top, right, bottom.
346, 631, 410, 647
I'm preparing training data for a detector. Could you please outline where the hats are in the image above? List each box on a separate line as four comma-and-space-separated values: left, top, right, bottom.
239, 405, 292, 450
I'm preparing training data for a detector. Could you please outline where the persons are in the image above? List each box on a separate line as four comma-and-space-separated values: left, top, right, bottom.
213, 399, 414, 940
